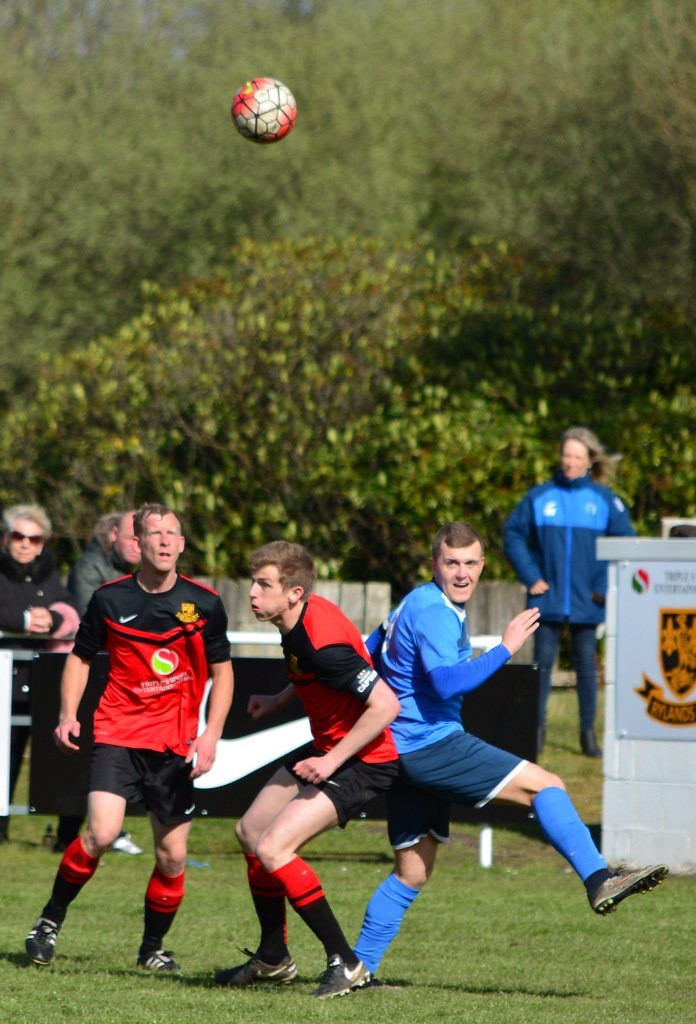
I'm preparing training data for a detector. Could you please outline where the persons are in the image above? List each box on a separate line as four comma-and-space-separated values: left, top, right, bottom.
0, 499, 79, 852
499, 426, 638, 759
26, 502, 234, 975
226, 541, 403, 1002
353, 521, 671, 984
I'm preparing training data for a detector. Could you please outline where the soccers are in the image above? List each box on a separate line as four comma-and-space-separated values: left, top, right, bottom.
231, 76, 298, 145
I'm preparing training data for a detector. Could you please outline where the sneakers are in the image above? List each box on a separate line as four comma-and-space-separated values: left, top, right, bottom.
136, 950, 181, 973
228, 945, 297, 984
312, 954, 372, 999
26, 918, 57, 965
589, 863, 669, 916
110, 834, 142, 855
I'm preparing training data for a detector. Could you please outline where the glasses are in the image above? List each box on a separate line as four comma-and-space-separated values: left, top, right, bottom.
10, 530, 42, 545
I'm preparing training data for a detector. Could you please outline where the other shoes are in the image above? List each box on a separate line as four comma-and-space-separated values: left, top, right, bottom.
580, 731, 602, 758
56, 839, 68, 851
537, 729, 546, 753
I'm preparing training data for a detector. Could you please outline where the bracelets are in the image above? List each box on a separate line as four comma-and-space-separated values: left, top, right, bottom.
23, 609, 32, 631
